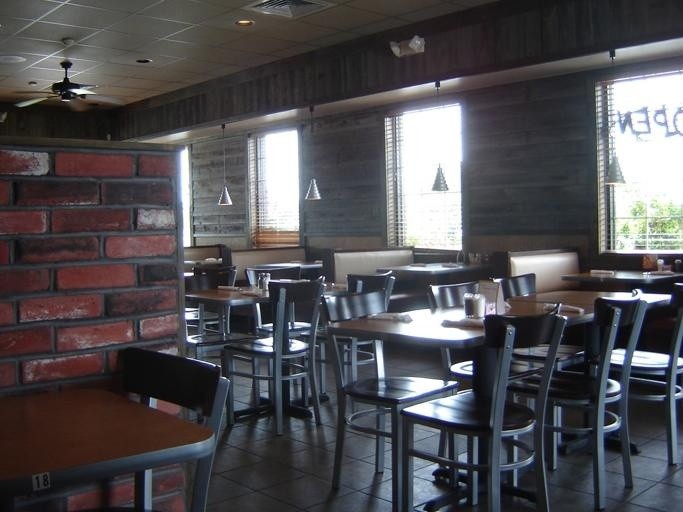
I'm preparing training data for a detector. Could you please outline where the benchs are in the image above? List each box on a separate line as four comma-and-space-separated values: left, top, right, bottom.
505, 247, 626, 341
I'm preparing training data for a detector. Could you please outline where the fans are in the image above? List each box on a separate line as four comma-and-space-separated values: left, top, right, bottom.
10, 38, 130, 109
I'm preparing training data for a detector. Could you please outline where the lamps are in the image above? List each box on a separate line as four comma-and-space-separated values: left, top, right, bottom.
300, 103, 326, 201
603, 40, 625, 187
216, 124, 232, 207
427, 79, 453, 196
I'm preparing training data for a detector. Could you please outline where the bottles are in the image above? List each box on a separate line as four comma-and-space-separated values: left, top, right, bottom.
469, 253, 476, 265
257, 272, 271, 291
658, 259, 664, 272
464, 293, 475, 318
475, 253, 483, 264
473, 293, 485, 319
456, 248, 465, 263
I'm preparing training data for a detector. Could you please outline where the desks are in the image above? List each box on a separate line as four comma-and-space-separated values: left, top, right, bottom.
376, 262, 500, 285
248, 261, 326, 280
0, 386, 215, 511
324, 287, 671, 511
333, 298, 569, 433
561, 261, 681, 314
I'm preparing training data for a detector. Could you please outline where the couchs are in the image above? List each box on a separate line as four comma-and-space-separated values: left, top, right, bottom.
228, 249, 306, 283
183, 245, 221, 260
333, 249, 415, 285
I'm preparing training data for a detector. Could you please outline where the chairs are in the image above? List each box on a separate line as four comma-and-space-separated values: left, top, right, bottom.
401, 313, 567, 512
494, 273, 591, 472
426, 277, 538, 473
589, 280, 682, 465
347, 275, 397, 306
115, 344, 232, 511
182, 266, 326, 433
508, 297, 650, 509
317, 287, 460, 511
